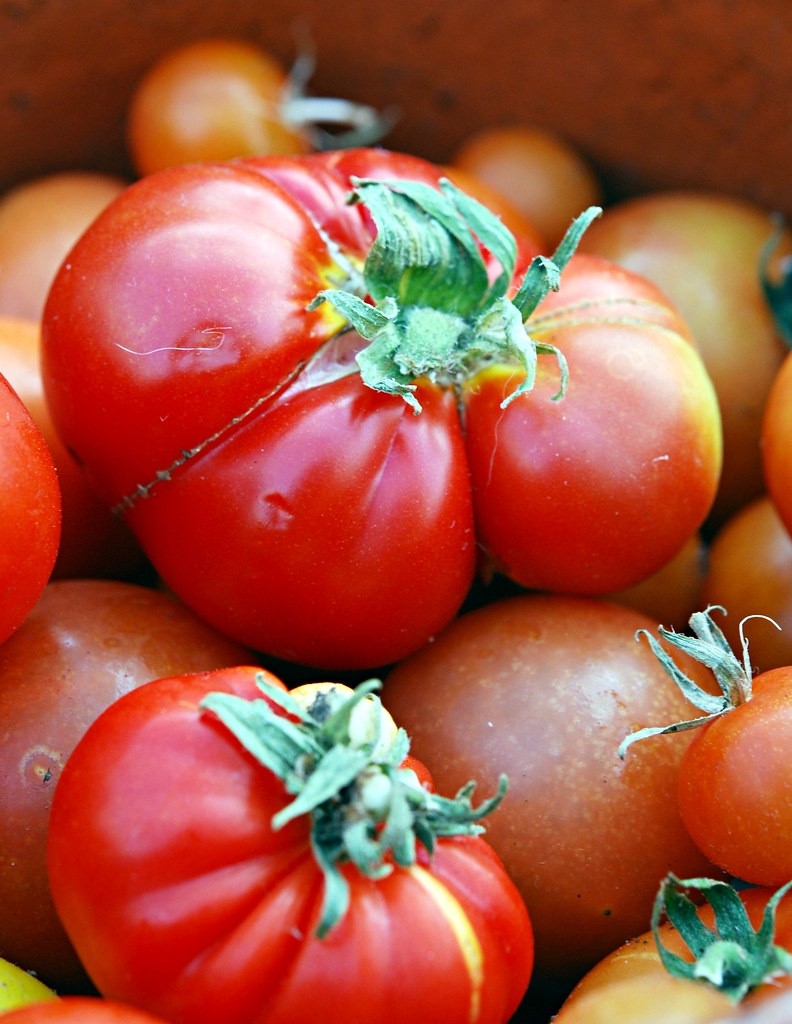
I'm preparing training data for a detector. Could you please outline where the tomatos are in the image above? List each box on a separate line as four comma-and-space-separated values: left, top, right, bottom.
0, 35, 792, 1024
